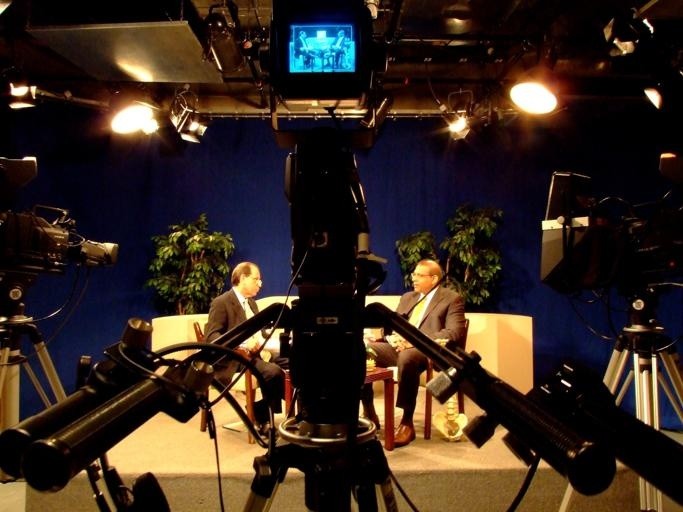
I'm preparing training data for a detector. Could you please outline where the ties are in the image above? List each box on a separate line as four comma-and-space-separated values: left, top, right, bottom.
244, 300, 254, 321
407, 298, 426, 324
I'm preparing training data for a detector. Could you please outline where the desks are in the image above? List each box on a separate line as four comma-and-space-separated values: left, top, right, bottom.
277, 358, 395, 454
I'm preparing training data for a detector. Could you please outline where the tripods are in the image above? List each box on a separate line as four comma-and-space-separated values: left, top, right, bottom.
554, 319, 683, 512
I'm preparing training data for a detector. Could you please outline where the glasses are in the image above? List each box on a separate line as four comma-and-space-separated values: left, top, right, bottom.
410, 272, 431, 278
248, 277, 263, 287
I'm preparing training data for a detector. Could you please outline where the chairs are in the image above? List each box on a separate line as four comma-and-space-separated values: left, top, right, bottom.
374, 320, 469, 447
188, 322, 280, 445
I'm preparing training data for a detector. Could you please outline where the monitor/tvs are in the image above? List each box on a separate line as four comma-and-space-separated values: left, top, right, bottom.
283, 18, 360, 84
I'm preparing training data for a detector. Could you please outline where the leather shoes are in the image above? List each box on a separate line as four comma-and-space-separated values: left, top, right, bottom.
393, 422, 415, 446
258, 421, 278, 439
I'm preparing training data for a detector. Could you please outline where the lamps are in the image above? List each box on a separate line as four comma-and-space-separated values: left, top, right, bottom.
442, 94, 497, 142
504, 40, 562, 117
167, 100, 208, 145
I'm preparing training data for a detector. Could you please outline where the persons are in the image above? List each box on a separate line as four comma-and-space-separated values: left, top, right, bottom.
358, 258, 467, 452
323, 29, 346, 69
292, 29, 315, 66
197, 260, 290, 448
